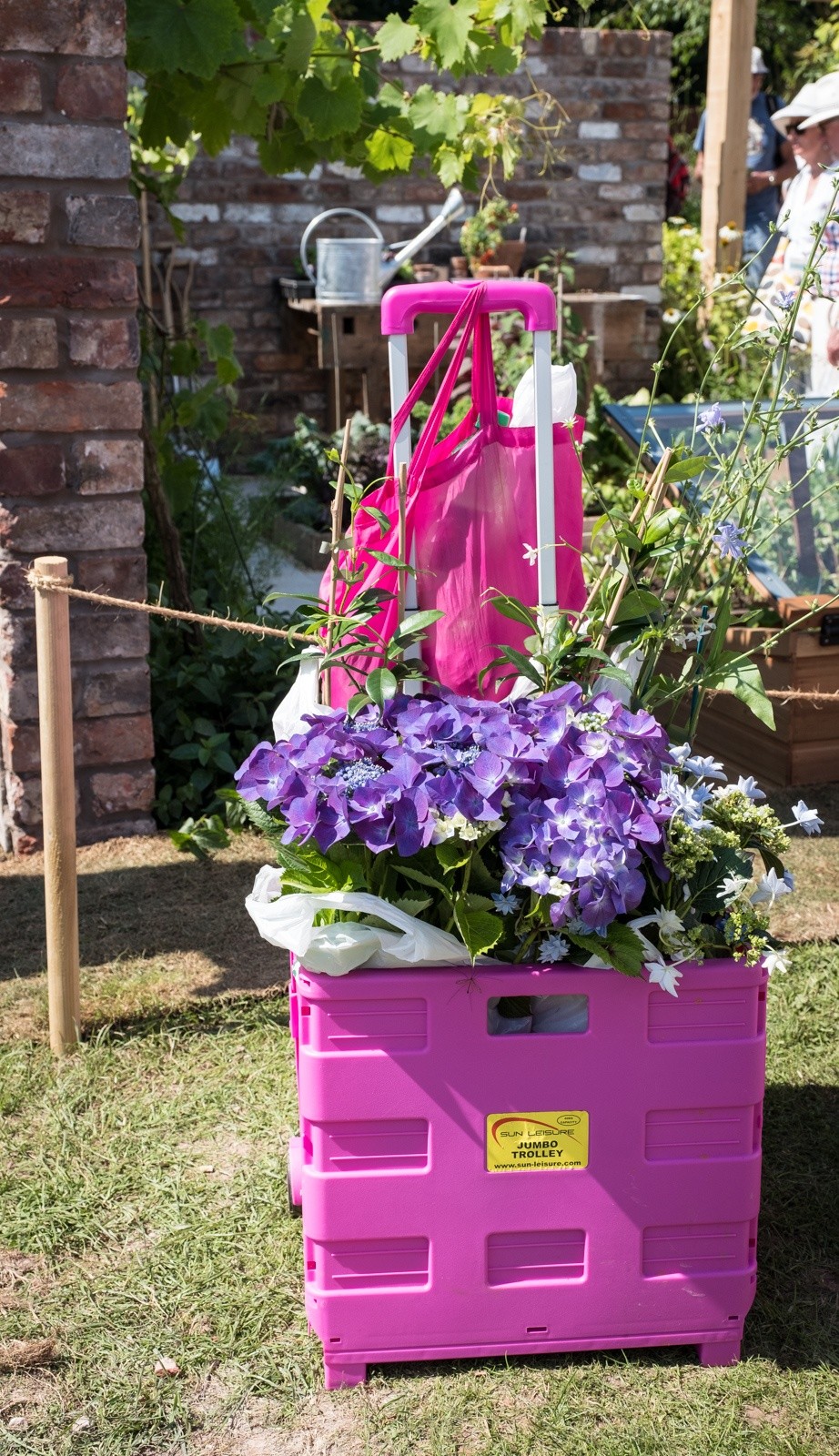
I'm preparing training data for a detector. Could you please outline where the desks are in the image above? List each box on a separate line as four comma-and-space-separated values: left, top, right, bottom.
289, 294, 646, 429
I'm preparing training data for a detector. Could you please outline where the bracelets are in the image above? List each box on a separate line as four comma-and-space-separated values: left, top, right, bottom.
765, 170, 777, 188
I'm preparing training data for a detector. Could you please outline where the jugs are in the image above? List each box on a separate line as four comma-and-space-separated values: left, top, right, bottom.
300, 189, 466, 302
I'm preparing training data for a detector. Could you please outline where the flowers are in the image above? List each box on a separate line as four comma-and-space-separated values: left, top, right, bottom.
459, 198, 520, 270
169, 405, 824, 997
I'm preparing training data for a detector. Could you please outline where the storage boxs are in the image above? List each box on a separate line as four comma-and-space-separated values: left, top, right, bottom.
287, 945, 770, 1391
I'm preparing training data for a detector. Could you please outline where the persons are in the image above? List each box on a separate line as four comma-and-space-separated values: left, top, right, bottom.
692, 48, 839, 476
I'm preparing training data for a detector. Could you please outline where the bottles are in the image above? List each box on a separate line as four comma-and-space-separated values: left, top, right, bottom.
423, 409, 510, 588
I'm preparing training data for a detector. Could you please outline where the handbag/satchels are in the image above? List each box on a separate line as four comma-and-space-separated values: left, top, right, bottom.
320, 298, 587, 716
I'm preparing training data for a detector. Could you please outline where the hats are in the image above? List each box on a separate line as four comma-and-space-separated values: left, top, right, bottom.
770, 83, 815, 140
749, 46, 770, 75
799, 70, 839, 131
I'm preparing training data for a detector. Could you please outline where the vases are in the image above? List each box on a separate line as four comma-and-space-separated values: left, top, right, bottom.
467, 240, 526, 278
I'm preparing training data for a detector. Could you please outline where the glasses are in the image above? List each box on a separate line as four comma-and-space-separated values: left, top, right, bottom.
786, 121, 806, 137
